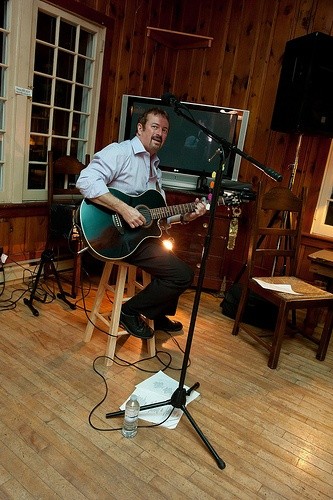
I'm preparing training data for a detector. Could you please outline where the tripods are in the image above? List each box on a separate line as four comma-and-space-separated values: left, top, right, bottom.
106, 109, 284, 470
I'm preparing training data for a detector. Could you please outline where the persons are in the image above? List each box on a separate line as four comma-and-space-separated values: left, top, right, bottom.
175, 112, 210, 172
76, 109, 206, 338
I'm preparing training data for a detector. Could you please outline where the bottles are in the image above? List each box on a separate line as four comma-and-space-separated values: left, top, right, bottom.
122, 394, 140, 438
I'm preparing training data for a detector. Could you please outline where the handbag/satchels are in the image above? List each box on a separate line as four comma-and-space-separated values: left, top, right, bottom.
220, 283, 279, 331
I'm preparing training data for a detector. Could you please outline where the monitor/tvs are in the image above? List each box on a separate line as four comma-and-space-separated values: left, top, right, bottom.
119, 94, 250, 191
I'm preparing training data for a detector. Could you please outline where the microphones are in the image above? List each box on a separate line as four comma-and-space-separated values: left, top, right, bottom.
161, 93, 189, 110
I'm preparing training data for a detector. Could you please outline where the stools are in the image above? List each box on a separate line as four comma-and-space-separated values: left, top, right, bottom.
83, 259, 156, 366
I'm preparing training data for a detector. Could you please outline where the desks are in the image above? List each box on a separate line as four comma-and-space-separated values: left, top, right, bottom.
303, 250, 333, 345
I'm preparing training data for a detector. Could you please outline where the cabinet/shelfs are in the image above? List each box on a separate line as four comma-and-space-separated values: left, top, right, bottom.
161, 188, 257, 293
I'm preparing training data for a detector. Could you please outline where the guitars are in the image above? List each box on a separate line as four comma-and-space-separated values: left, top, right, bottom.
76, 187, 257, 263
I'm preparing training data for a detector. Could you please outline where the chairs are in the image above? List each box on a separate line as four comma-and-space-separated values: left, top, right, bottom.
46, 151, 90, 297
232, 181, 333, 369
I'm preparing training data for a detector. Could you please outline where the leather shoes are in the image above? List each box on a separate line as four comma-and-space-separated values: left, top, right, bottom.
119, 312, 154, 339
154, 315, 182, 331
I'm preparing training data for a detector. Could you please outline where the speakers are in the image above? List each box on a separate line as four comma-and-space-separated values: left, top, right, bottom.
271, 31, 333, 139
